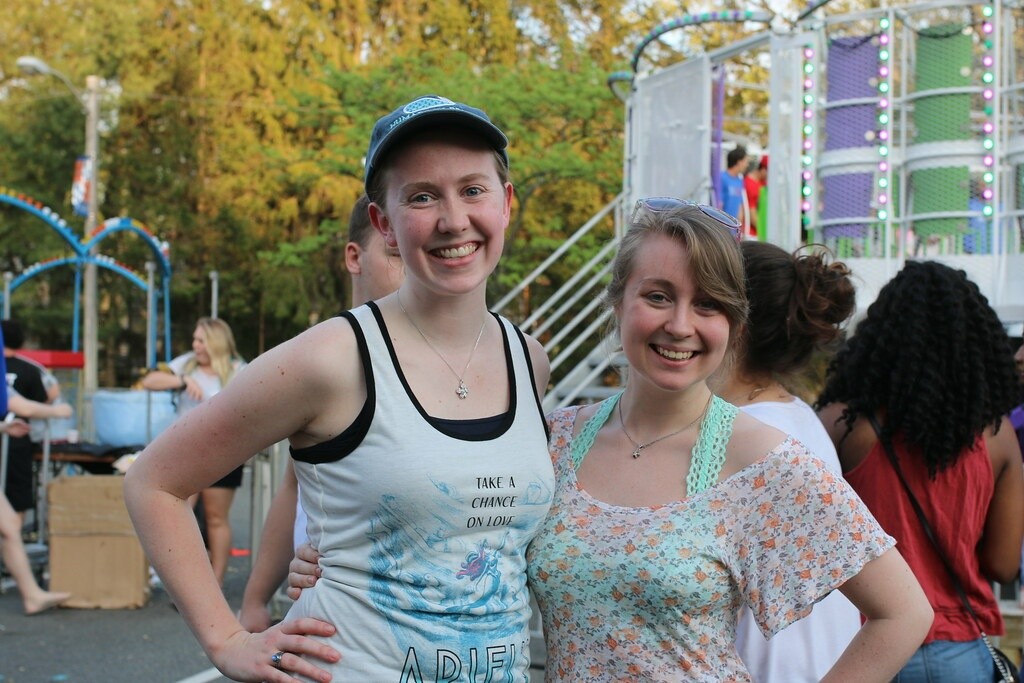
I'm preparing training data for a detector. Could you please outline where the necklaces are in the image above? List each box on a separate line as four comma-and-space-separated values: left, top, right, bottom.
618, 388, 706, 458
397, 283, 487, 399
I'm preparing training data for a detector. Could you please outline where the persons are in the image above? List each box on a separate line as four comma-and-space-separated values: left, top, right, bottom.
741, 154, 770, 236
122, 92, 558, 683
813, 260, 1023, 682
15, 353, 61, 445
0, 319, 50, 532
286, 197, 934, 683
721, 147, 749, 236
705, 236, 936, 682
140, 318, 249, 595
0, 382, 72, 616
237, 197, 406, 635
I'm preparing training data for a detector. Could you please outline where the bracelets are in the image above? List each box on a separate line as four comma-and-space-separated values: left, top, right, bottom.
181, 375, 186, 387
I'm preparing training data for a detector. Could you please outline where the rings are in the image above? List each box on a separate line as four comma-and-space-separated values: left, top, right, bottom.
272, 652, 284, 668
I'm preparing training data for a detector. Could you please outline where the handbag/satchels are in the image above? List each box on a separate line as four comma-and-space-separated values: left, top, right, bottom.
990, 643, 1020, 683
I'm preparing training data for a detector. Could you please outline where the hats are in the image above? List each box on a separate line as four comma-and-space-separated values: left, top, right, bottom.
761, 156, 768, 167
364, 95, 509, 203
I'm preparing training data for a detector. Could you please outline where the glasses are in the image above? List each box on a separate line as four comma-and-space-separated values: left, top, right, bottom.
629, 196, 742, 245
